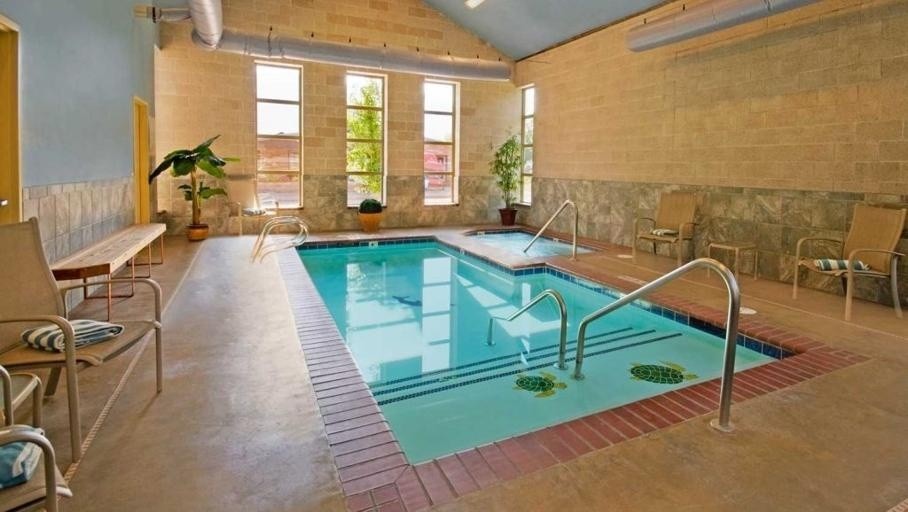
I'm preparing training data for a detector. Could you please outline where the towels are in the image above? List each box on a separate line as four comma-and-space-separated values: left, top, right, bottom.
651, 225, 678, 238
813, 259, 871, 271
20, 319, 125, 353
1, 426, 45, 489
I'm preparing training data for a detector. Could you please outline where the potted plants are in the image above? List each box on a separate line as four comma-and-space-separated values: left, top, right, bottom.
148, 134, 241, 242
357, 199, 383, 234
487, 134, 528, 226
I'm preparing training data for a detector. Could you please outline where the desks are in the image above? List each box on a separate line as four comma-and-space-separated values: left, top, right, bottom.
707, 241, 760, 279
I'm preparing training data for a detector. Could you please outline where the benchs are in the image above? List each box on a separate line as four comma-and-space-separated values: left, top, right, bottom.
57, 222, 167, 321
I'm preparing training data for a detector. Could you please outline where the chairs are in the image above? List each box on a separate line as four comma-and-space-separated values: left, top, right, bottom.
0, 367, 71, 511
632, 192, 699, 267
792, 203, 908, 322
0, 217, 163, 463
